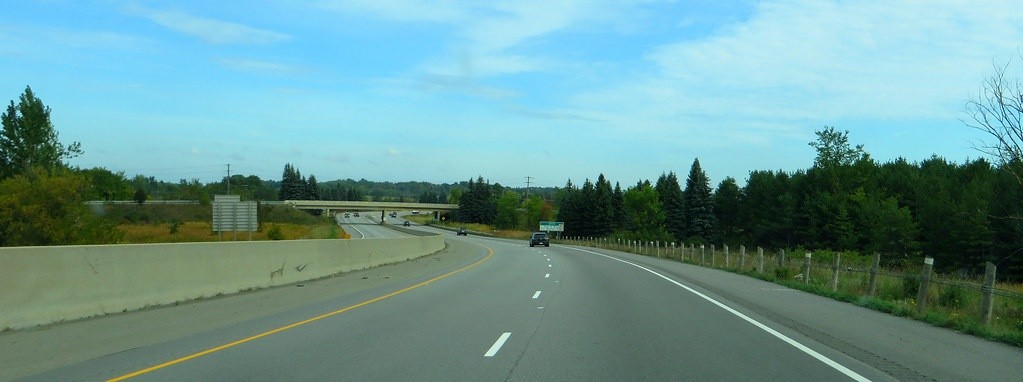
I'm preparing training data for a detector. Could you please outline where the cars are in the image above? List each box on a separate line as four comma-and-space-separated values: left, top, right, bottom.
529, 232, 550, 247
344, 212, 359, 218
404, 221, 410, 226
389, 212, 397, 218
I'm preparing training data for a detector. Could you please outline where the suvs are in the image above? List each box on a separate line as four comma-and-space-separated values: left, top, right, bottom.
457, 227, 467, 236
